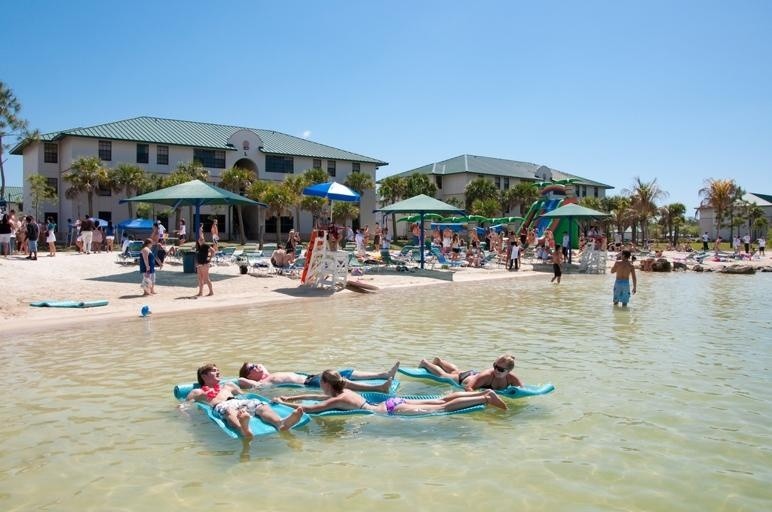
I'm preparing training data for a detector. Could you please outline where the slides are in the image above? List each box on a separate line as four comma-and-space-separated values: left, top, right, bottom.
516, 199, 564, 245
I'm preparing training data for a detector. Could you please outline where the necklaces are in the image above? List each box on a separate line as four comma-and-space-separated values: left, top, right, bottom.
262, 373, 268, 380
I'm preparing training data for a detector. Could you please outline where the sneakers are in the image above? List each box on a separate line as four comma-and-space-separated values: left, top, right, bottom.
26, 256, 32, 259
30, 256, 37, 260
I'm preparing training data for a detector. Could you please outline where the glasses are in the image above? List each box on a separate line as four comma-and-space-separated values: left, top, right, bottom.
492, 362, 511, 374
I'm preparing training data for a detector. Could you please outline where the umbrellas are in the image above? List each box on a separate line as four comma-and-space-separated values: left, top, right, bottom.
538, 203, 612, 264
117, 180, 270, 245
371, 193, 468, 273
301, 180, 359, 226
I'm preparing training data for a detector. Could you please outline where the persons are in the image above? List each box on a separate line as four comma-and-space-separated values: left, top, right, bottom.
172, 361, 304, 441
194, 235, 216, 296
150, 230, 164, 295
140, 239, 157, 296
269, 368, 508, 415
609, 250, 636, 307
550, 243, 564, 283
237, 359, 401, 392
0, 208, 767, 276
418, 352, 526, 394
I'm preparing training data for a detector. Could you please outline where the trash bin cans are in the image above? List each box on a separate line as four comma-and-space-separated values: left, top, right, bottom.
183, 251, 197, 273
